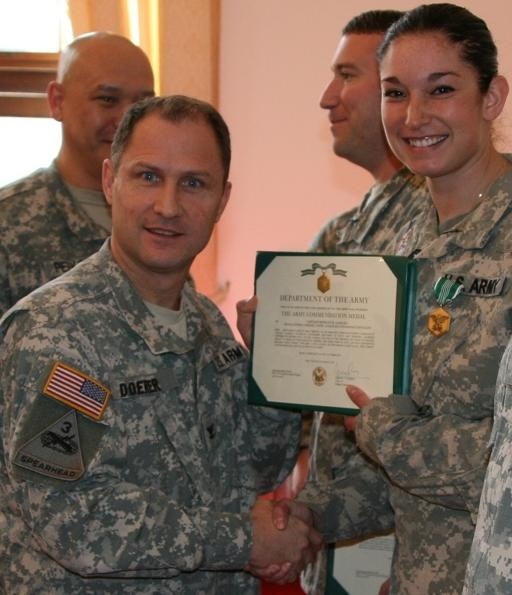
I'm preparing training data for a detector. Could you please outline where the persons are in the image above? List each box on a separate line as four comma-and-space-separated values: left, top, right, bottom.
236, 9, 413, 349
2, 94, 323, 594
1, 28, 195, 319
247, 2, 510, 594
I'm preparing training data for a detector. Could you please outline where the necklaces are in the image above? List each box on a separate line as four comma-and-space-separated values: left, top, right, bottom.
474, 160, 509, 209
